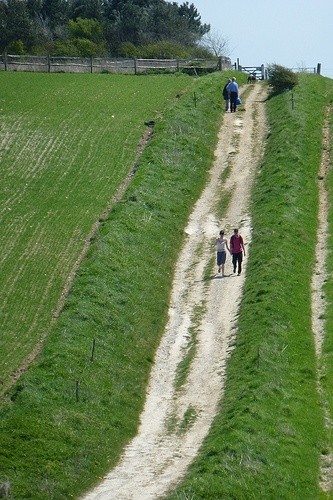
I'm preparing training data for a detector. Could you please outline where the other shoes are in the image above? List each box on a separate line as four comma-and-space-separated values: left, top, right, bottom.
233, 269, 236, 273
237, 273, 240, 276
218, 268, 221, 272
222, 275, 225, 277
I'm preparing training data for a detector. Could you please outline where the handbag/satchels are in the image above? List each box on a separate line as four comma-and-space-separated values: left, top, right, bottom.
235, 97, 241, 105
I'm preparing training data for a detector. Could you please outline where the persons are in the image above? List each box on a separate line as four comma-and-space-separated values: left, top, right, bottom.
210, 230, 231, 277
229, 228, 246, 276
222, 78, 234, 112
226, 76, 240, 113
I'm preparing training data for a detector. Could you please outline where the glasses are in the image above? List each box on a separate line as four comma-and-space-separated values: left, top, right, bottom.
220, 233, 224, 235
